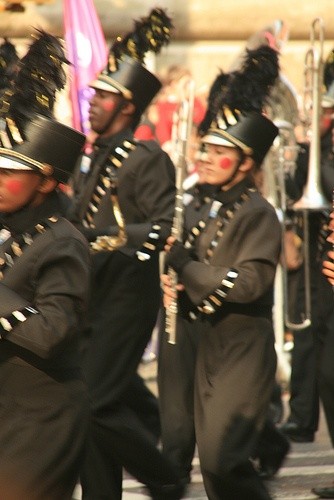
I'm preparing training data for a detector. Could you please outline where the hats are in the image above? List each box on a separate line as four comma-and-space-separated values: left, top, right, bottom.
200, 45, 281, 158
89, 9, 174, 103
0, 25, 86, 184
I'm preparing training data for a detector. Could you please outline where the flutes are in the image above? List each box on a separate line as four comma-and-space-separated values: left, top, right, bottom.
164, 101, 193, 345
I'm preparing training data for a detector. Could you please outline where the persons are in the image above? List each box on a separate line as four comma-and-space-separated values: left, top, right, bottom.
161, 104, 285, 500
280, 46, 334, 500
0, 100, 95, 500
154, 70, 291, 500
276, 105, 334, 446
72, 56, 178, 499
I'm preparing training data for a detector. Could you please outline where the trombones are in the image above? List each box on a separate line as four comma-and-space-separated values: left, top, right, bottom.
274, 18, 332, 332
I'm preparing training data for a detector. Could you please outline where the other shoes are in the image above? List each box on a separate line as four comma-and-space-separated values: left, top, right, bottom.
281, 422, 314, 442
258, 440, 291, 480
311, 486, 334, 499
